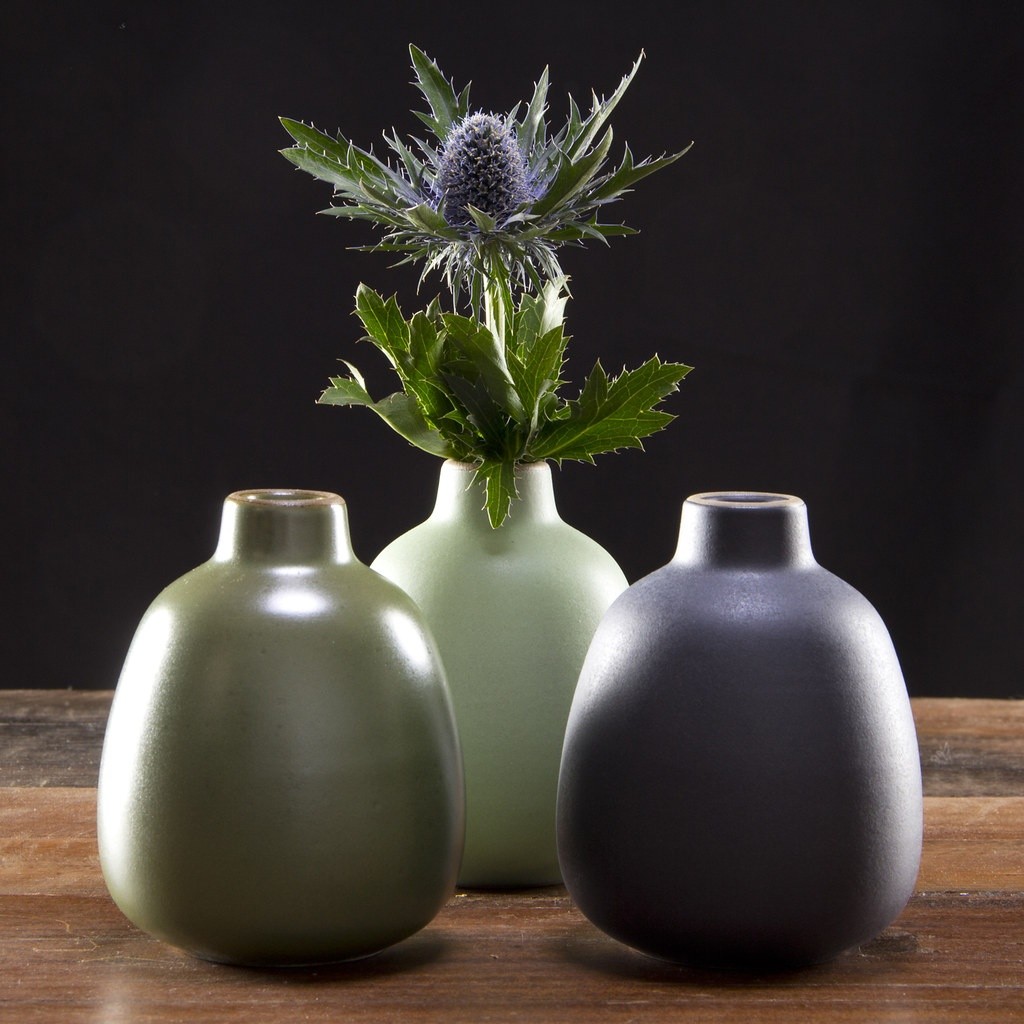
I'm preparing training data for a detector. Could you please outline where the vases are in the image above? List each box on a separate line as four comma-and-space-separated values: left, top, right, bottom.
372, 462, 628, 891
552, 488, 923, 965
97, 489, 464, 968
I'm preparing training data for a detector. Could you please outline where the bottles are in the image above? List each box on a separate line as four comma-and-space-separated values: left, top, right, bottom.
97, 483, 468, 978
550, 485, 926, 974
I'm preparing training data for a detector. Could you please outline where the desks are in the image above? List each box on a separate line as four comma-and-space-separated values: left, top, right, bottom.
0, 686, 1024, 1024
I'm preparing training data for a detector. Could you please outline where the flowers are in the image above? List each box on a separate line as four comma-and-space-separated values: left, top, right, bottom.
275, 41, 691, 531
